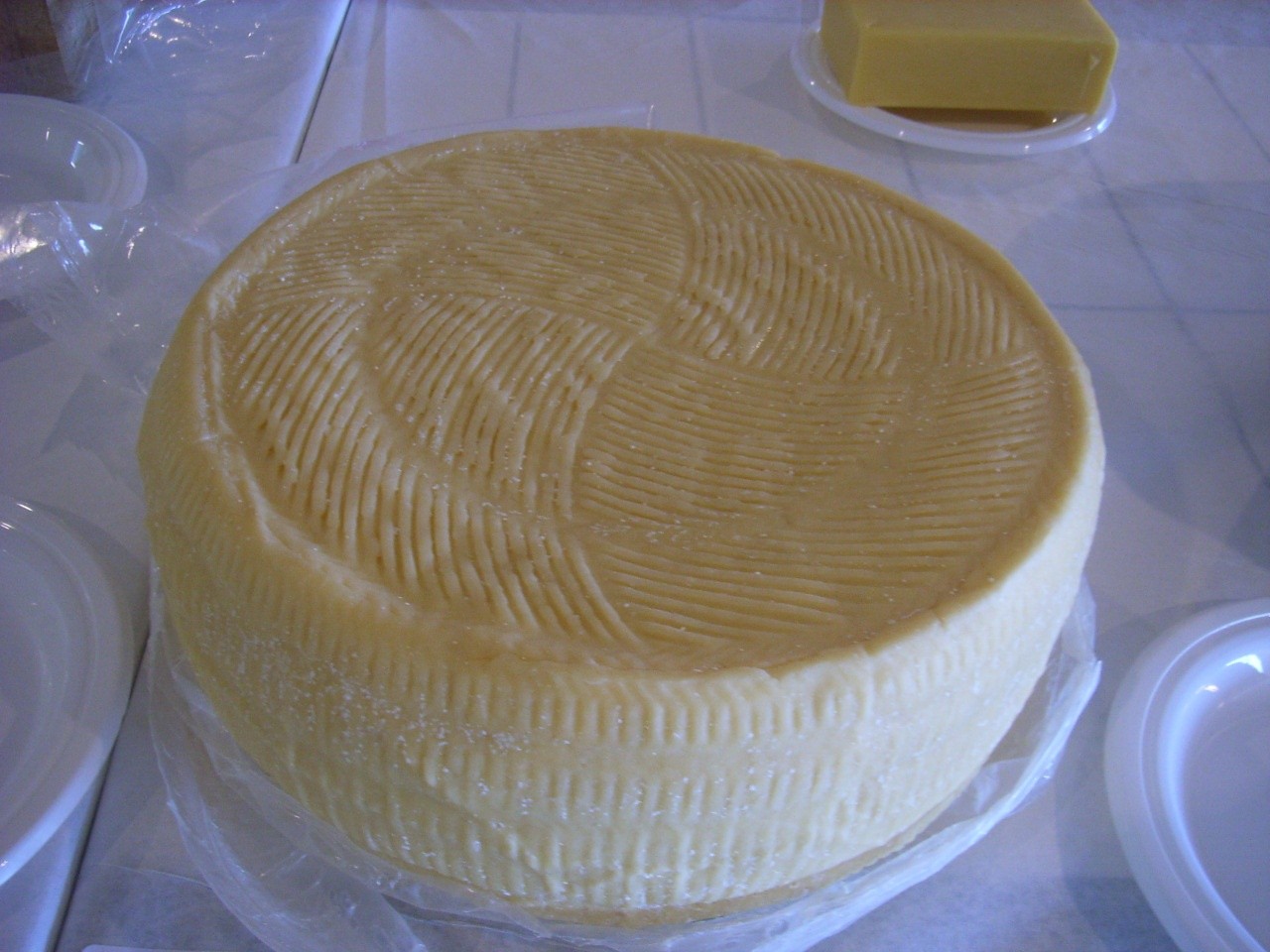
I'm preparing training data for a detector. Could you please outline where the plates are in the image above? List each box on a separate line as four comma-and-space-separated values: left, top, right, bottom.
789, 14, 1119, 160
1, 493, 128, 893
0, 84, 152, 305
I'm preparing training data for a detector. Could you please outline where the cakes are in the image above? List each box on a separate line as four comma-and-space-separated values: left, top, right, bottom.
116, 127, 1109, 917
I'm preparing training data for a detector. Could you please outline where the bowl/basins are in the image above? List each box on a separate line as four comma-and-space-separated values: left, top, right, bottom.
1100, 598, 1268, 952
